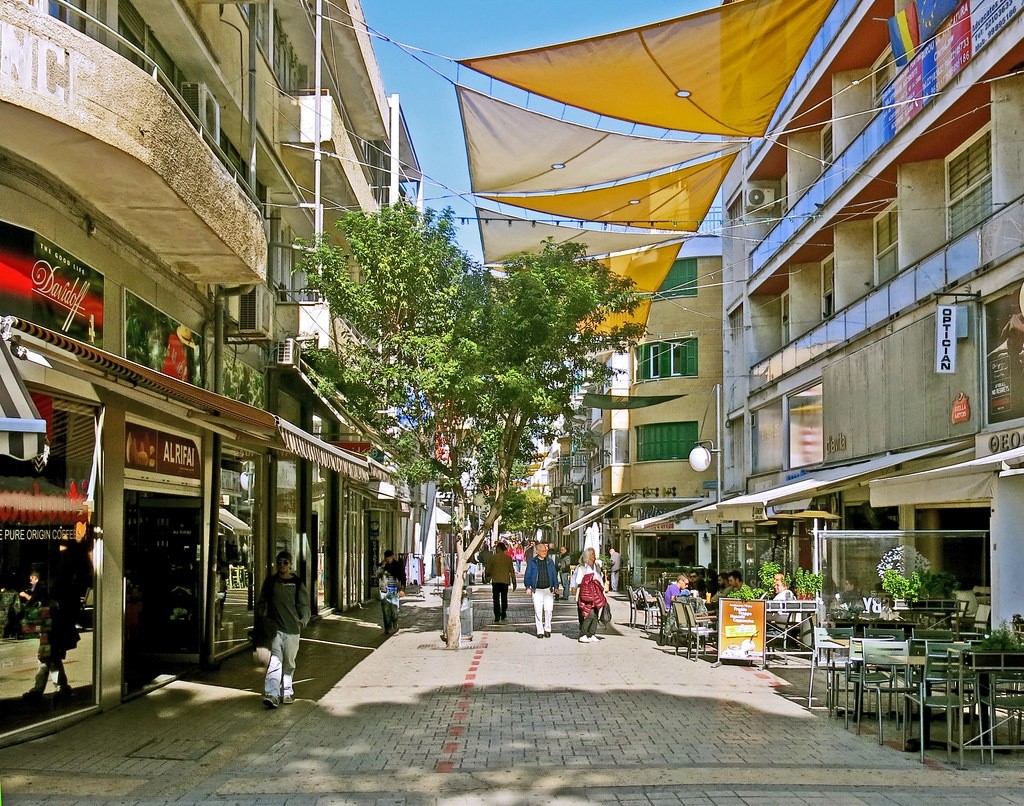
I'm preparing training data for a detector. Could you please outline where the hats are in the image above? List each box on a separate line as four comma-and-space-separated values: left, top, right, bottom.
384, 550, 393, 557
277, 551, 292, 560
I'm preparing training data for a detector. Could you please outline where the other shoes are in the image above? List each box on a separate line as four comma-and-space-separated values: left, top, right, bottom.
501, 613, 506, 620
537, 634, 543, 638
494, 616, 500, 622
579, 635, 589, 643
559, 596, 568, 600
544, 631, 550, 638
589, 634, 600, 642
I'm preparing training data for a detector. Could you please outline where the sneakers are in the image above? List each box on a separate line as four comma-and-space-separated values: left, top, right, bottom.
283, 694, 293, 704
263, 695, 279, 707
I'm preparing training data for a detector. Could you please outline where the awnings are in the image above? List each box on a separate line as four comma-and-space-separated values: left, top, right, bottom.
218, 505, 250, 536
692, 503, 730, 523
12, 317, 372, 482
868, 444, 1024, 508
561, 493, 633, 535
334, 446, 392, 481
715, 439, 975, 524
626, 492, 739, 529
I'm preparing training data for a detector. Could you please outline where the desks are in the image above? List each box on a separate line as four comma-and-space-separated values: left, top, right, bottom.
816, 639, 850, 717
849, 656, 959, 735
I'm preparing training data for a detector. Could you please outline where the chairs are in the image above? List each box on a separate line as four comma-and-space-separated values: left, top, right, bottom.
808, 626, 1024, 770
626, 585, 718, 661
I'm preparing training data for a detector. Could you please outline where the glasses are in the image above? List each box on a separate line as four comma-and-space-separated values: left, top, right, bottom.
682, 580, 688, 586
277, 560, 291, 565
689, 575, 698, 578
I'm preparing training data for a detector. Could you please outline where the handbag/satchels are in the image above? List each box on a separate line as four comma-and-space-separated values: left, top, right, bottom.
636, 597, 647, 610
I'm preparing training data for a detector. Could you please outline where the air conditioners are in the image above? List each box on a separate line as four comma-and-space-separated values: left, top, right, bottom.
223, 283, 273, 343
180, 81, 221, 155
745, 188, 774, 212
263, 338, 301, 375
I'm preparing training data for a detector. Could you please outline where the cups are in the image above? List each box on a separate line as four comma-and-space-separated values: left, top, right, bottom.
706, 593, 711, 602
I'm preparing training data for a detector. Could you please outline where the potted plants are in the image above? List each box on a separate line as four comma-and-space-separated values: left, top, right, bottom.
792, 566, 824, 600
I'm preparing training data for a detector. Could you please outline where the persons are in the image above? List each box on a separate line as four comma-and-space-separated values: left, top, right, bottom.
771, 571, 802, 622
569, 546, 605, 643
19, 568, 83, 697
525, 542, 559, 640
833, 577, 860, 601
608, 548, 623, 592
688, 571, 705, 592
709, 572, 754, 602
478, 537, 571, 601
663, 575, 691, 611
254, 550, 312, 709
374, 549, 406, 634
484, 541, 518, 623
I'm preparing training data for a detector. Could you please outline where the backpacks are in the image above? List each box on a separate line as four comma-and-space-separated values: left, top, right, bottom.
599, 602, 612, 623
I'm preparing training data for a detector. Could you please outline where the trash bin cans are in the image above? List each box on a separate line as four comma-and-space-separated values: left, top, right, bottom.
443, 586, 474, 642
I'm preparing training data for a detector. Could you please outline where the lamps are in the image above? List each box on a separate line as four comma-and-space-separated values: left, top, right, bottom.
689, 439, 722, 472
